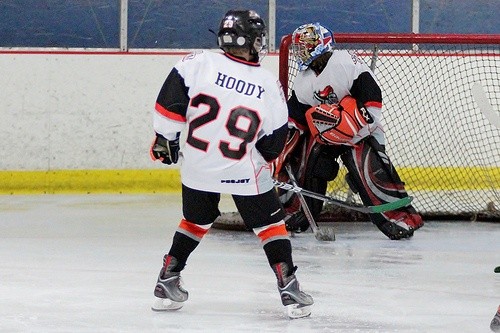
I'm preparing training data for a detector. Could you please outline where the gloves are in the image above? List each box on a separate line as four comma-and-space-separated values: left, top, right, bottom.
150, 132, 180, 165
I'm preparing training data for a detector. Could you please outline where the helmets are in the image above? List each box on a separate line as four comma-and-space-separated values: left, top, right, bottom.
216, 8, 267, 63
291, 21, 336, 71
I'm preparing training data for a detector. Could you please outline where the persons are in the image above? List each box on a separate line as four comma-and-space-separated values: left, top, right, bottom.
268, 23, 424, 240
150, 8, 314, 319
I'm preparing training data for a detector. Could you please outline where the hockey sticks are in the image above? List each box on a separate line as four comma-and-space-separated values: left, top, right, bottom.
178, 151, 414, 214
282, 163, 336, 241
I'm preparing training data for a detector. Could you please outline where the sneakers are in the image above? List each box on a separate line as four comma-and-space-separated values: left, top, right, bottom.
151, 254, 188, 311
271, 261, 314, 319
